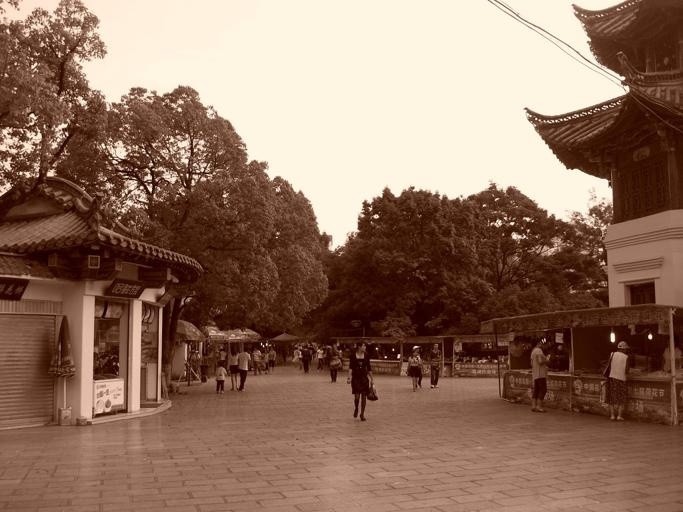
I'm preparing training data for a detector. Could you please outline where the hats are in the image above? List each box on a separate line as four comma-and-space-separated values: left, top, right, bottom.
413, 346, 421, 350
617, 341, 630, 350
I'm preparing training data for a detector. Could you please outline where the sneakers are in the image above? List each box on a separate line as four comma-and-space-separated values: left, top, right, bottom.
611, 414, 624, 421
353, 409, 366, 421
532, 407, 547, 414
412, 385, 439, 393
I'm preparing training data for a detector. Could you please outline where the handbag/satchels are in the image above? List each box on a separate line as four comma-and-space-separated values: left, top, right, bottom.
368, 383, 378, 402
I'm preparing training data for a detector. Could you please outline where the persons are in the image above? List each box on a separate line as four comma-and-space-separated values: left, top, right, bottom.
346, 341, 375, 420
407, 350, 419, 392
412, 346, 425, 388
661, 336, 680, 372
530, 338, 553, 412
215, 342, 344, 393
429, 343, 442, 388
602, 340, 631, 421
